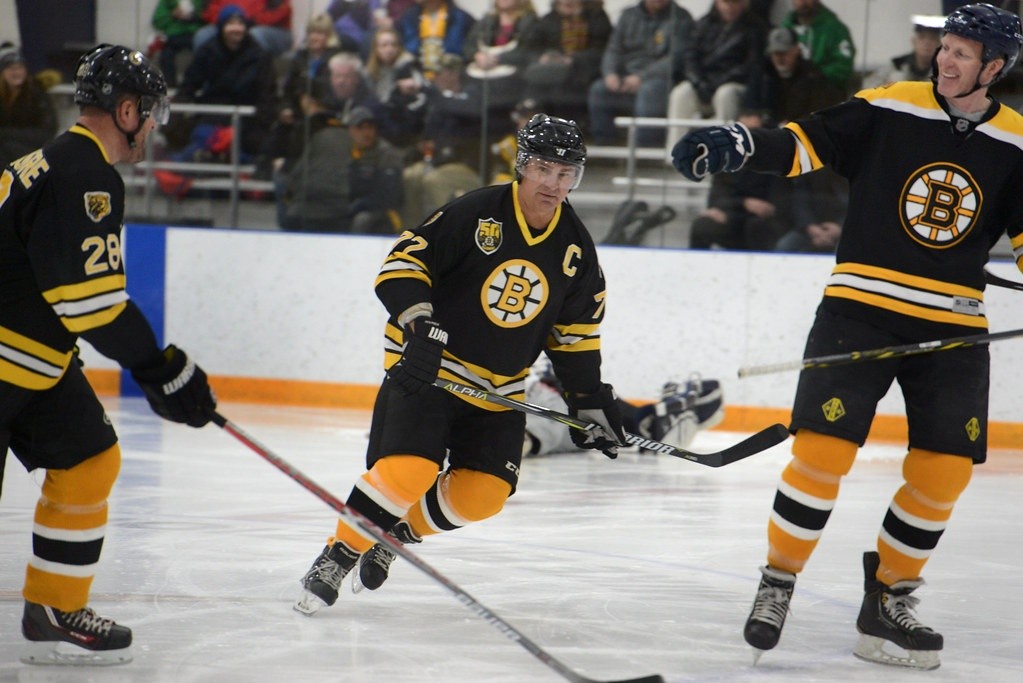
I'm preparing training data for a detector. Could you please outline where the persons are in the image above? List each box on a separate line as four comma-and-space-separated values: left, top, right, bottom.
0, 53, 59, 174
672, 0, 1023, 670
144, 0, 1023, 254
522, 354, 723, 456
0, 41, 214, 665
294, 113, 630, 616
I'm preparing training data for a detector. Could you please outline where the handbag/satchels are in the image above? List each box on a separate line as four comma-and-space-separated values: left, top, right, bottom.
466, 37, 521, 81
520, 51, 578, 89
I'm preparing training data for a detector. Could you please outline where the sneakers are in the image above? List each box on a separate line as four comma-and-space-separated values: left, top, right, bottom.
295, 536, 363, 616
350, 521, 423, 593
746, 561, 797, 670
849, 552, 944, 670
20, 598, 133, 666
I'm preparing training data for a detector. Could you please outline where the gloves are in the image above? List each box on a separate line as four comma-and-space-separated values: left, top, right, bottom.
135, 344, 216, 429
671, 122, 757, 183
385, 316, 451, 407
563, 383, 631, 458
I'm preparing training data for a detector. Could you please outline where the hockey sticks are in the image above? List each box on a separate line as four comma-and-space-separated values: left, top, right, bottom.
433, 376, 790, 470
736, 326, 1023, 380
210, 411, 666, 683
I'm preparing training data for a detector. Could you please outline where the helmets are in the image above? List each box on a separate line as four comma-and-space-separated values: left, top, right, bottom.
942, 3, 1023, 87
72, 43, 169, 113
514, 112, 585, 178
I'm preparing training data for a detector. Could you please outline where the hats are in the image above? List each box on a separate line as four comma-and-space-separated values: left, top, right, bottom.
0, 42, 28, 71
911, 13, 950, 33
349, 106, 376, 127
764, 27, 799, 53
217, 6, 247, 29
